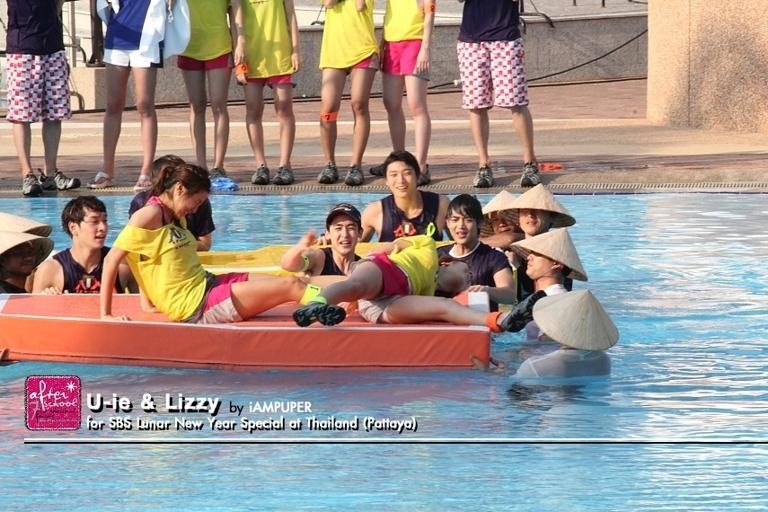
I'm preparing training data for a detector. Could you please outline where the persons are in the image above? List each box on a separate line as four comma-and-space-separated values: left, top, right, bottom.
229, 0, 300, 185
317, 0, 382, 186
0, 150, 619, 412
6, 0, 80, 195
86, 0, 191, 191
177, 0, 247, 179
369, 0, 436, 186
456, 0, 542, 187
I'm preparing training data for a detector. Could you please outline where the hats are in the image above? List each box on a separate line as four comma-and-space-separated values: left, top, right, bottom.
482, 181, 577, 228
325, 202, 362, 232
531, 289, 620, 352
509, 228, 589, 283
0, 209, 54, 275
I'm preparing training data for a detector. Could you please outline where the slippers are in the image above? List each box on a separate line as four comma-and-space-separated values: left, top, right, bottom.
133, 174, 154, 191
86, 171, 115, 189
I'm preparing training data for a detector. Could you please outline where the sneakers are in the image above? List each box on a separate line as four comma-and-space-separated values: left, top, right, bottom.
251, 163, 270, 186
500, 290, 547, 333
38, 168, 82, 191
473, 165, 495, 188
21, 173, 42, 196
519, 161, 542, 186
273, 166, 295, 185
209, 168, 240, 192
293, 303, 347, 328
417, 164, 434, 187
316, 162, 387, 185
